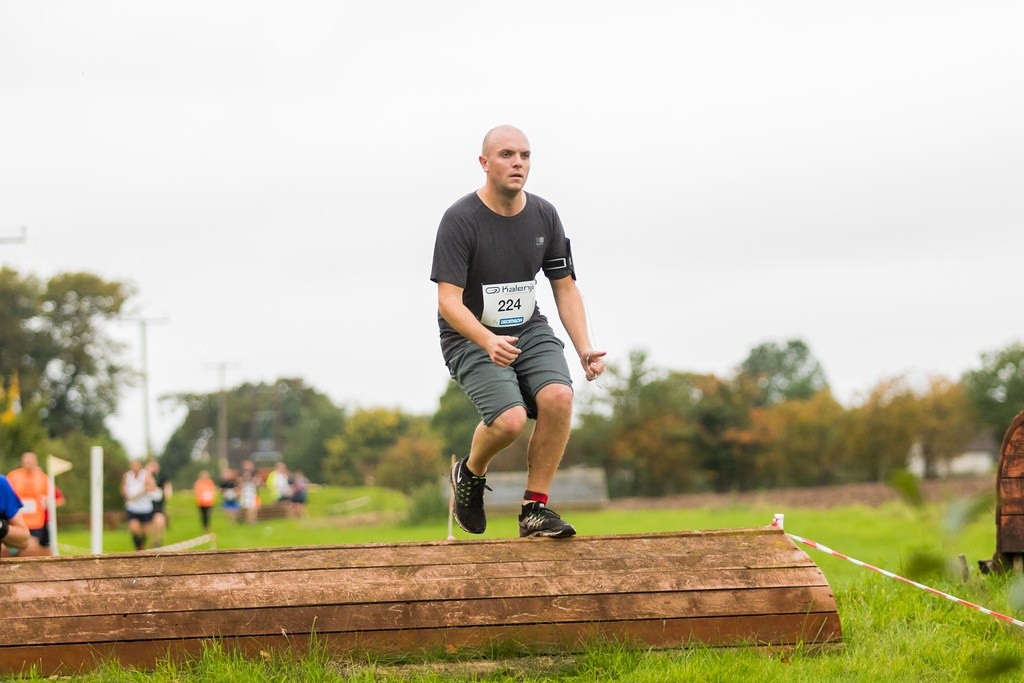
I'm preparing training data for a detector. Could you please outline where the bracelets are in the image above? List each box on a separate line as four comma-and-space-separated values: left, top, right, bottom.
0, 519, 9, 540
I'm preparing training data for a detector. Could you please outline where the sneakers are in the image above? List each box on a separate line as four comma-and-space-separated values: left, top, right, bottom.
518, 502, 576, 538
450, 456, 486, 533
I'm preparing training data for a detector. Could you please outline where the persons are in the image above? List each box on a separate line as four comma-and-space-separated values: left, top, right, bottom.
120, 458, 162, 552
143, 460, 173, 547
7, 452, 50, 557
429, 126, 607, 537
0, 475, 29, 558
40, 485, 67, 547
194, 468, 216, 529
220, 460, 310, 525
